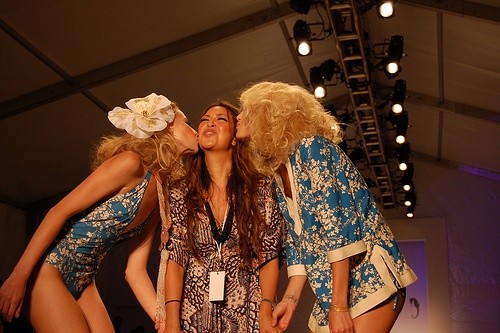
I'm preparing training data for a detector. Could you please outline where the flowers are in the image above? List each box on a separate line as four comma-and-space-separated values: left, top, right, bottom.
108, 94, 175, 140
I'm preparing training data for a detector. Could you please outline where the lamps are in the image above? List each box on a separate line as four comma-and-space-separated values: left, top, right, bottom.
289, 0, 418, 220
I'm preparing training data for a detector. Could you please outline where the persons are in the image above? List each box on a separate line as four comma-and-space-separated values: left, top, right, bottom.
236, 82, 417, 333
164, 102, 288, 333
0, 94, 199, 333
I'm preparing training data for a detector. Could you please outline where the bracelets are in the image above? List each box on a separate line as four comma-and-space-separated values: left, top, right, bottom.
261, 299, 276, 310
330, 304, 349, 312
165, 300, 180, 305
284, 296, 298, 304
